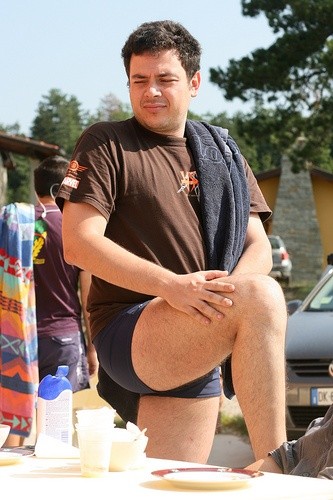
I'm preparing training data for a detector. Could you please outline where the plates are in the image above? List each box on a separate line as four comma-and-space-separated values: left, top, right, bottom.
0, 451, 22, 466
150, 466, 264, 490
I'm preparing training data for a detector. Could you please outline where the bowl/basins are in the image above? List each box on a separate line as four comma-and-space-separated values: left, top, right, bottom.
106, 433, 147, 471
0, 425, 10, 449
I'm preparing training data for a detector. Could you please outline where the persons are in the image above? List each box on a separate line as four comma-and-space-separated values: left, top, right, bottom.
53, 18, 289, 473
24, 158, 100, 403
243, 355, 333, 483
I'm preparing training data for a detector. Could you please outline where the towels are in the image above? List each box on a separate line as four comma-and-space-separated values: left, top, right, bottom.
183, 117, 252, 400
0, 201, 39, 437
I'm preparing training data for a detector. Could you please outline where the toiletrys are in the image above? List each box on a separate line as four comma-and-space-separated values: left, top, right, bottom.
33, 365, 73, 457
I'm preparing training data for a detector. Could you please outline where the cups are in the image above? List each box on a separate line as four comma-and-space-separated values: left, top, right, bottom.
73, 405, 115, 478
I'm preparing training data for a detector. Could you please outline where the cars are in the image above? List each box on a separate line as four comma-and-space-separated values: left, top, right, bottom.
263, 235, 292, 286
282, 253, 333, 442
